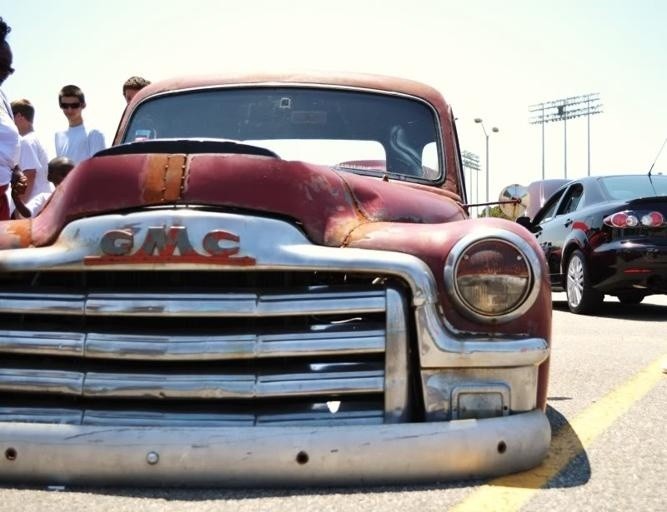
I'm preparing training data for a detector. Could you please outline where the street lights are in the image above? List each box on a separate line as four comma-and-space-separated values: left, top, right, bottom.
475, 117, 499, 218
460, 149, 479, 221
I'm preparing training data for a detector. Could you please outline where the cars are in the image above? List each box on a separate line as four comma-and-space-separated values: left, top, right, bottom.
515, 138, 667, 313
2, 74, 555, 481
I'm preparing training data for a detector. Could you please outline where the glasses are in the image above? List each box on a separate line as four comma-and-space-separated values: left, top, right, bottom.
59, 102, 82, 109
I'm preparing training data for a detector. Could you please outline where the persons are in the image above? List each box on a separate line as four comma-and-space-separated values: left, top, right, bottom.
54, 83, 107, 170
11, 157, 76, 220
10, 101, 50, 203
121, 75, 151, 104
0, 19, 21, 224
